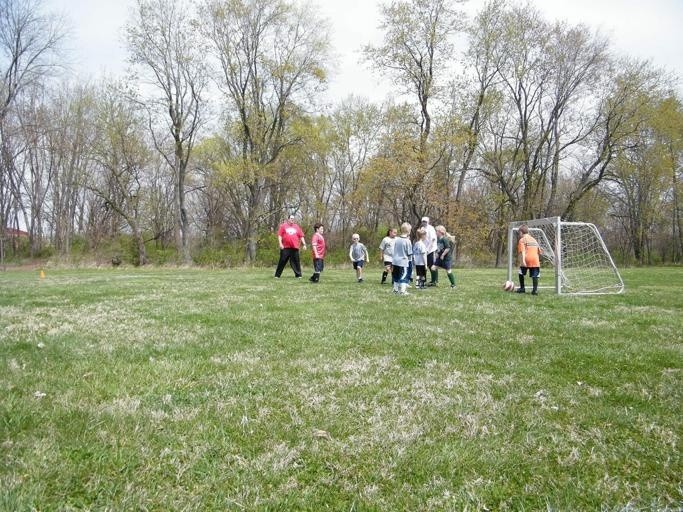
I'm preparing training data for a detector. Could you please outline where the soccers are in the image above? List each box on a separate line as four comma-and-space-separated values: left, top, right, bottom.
502, 281, 514, 292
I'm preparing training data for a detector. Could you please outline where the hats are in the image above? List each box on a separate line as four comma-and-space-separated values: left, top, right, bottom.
421, 216, 429, 222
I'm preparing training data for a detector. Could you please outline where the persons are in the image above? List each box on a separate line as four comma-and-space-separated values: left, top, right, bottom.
274, 215, 306, 279
310, 223, 326, 283
379, 217, 437, 295
425, 225, 456, 289
516, 224, 542, 296
349, 233, 369, 282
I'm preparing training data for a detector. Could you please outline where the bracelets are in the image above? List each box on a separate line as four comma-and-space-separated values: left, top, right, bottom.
302, 244, 306, 246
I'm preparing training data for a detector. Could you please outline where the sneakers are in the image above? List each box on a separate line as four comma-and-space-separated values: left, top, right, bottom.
380, 281, 458, 296
514, 288, 539, 296
357, 277, 365, 282
274, 276, 302, 279
309, 276, 319, 282
